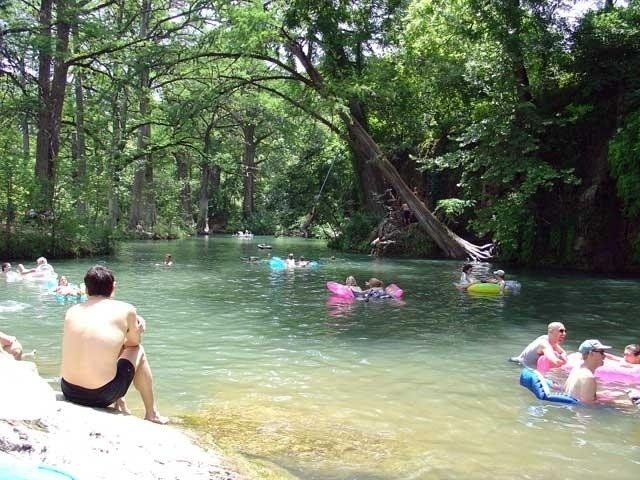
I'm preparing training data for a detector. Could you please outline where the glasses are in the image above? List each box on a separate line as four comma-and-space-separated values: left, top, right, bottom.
558, 329, 568, 333
592, 349, 605, 355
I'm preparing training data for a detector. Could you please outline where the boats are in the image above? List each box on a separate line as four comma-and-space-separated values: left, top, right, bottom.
467, 283, 499, 294
257, 243, 272, 249
232, 233, 254, 238
326, 281, 403, 300
520, 365, 619, 415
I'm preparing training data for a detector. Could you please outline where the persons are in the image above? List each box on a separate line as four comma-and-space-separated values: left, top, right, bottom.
512, 319, 640, 420
283, 250, 311, 270
459, 262, 510, 297
399, 186, 426, 225
0, 330, 38, 361
2, 256, 81, 299
235, 229, 253, 236
164, 254, 172, 266
342, 273, 389, 301
369, 235, 396, 258
60, 266, 170, 424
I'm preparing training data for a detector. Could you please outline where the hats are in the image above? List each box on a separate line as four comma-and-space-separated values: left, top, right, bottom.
578, 339, 613, 354
493, 270, 505, 277
